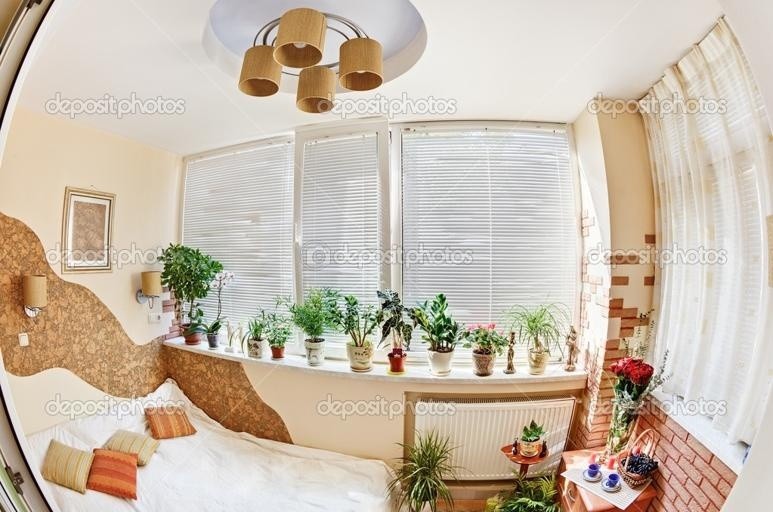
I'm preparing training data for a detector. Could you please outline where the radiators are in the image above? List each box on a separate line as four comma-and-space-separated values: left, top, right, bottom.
410, 393, 577, 482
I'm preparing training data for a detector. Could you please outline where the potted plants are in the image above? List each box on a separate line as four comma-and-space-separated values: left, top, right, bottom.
247, 322, 267, 358
331, 298, 382, 371
261, 297, 294, 358
387, 431, 458, 512
375, 289, 419, 374
290, 289, 337, 367
505, 300, 569, 374
156, 242, 218, 346
520, 421, 544, 459
407, 295, 465, 375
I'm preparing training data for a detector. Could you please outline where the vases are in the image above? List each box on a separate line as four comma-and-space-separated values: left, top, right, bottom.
472, 347, 497, 376
207, 333, 219, 348
605, 398, 637, 455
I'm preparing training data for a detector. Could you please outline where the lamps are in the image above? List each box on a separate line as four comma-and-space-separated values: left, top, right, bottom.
19, 273, 49, 319
233, 4, 384, 114
137, 270, 161, 310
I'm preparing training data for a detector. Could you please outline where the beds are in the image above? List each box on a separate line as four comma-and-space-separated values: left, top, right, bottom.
28, 378, 409, 511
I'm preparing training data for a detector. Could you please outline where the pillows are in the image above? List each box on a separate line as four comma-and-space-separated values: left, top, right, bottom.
144, 405, 197, 439
85, 447, 139, 502
38, 436, 93, 495
104, 430, 157, 467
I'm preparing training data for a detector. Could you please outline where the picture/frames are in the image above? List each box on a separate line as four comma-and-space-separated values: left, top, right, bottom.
59, 187, 117, 275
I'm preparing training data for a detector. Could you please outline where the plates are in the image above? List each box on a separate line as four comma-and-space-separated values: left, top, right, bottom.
601, 478, 621, 492
582, 469, 603, 482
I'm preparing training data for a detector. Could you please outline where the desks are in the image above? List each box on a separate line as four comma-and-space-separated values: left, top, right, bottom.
501, 443, 549, 482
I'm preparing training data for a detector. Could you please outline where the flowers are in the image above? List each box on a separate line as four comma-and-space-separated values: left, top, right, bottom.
460, 321, 509, 356
183, 270, 234, 337
600, 307, 670, 449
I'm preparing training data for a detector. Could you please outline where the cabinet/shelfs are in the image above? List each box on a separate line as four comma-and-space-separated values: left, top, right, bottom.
555, 445, 656, 512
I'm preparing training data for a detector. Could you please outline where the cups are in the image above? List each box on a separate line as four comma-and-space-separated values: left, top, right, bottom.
606, 474, 620, 487
588, 464, 601, 477
